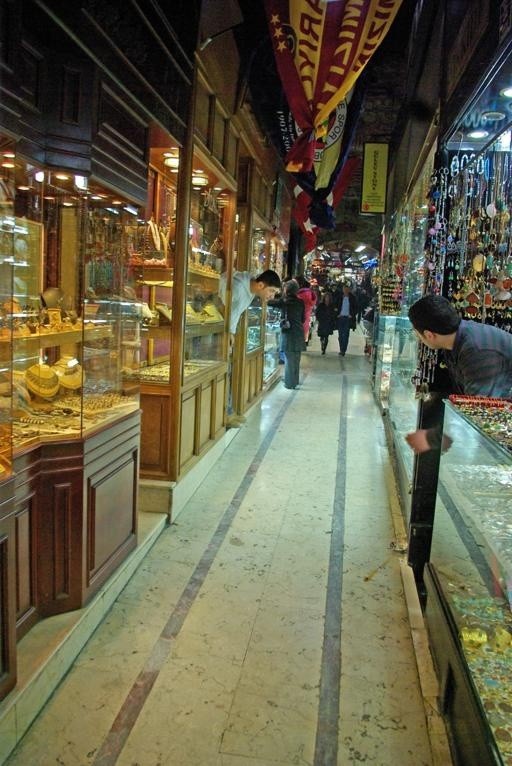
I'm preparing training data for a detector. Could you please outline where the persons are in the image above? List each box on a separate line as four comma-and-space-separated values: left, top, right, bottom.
404, 295, 512, 456
193, 268, 370, 429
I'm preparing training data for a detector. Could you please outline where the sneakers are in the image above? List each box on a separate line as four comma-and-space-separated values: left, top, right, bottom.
226, 415, 240, 427
232, 415, 245, 423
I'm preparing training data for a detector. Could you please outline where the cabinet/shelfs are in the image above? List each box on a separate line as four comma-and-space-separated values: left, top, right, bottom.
119, 206, 229, 483
422, 400, 512, 766
231, 211, 286, 416
369, 310, 443, 568
1, 151, 140, 702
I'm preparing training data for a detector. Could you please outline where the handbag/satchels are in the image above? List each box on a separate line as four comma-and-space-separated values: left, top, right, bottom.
279, 319, 290, 329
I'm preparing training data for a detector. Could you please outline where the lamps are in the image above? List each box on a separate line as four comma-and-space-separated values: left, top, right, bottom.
351, 241, 368, 254
